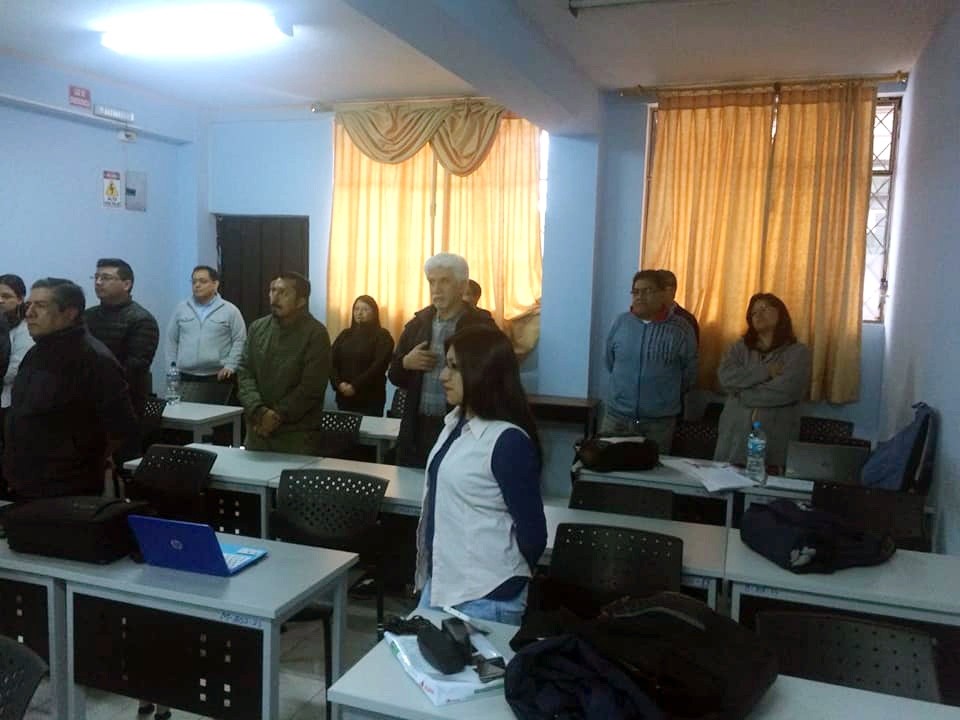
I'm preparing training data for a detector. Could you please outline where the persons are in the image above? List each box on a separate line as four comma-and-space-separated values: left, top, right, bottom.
601, 269, 704, 457
462, 280, 481, 306
329, 295, 394, 418
237, 272, 330, 444
714, 293, 809, 477
412, 326, 548, 627
0, 259, 159, 504
165, 266, 248, 446
389, 253, 499, 468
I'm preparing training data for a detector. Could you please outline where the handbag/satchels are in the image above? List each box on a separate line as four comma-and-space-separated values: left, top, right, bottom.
2, 494, 153, 563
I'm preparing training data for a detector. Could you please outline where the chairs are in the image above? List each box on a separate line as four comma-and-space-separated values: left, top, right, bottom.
0, 386, 944, 720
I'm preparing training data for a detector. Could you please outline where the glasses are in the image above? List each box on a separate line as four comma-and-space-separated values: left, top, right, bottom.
95, 275, 124, 281
630, 288, 659, 296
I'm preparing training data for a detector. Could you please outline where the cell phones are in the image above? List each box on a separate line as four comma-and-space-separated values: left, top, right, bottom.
477, 656, 507, 682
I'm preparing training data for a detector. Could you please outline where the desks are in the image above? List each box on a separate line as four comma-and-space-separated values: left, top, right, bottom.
524, 391, 601, 442
327, 612, 960, 720
268, 455, 427, 519
0, 500, 360, 720
157, 401, 246, 445
725, 526, 959, 625
741, 469, 813, 512
576, 456, 737, 525
531, 504, 726, 614
321, 413, 402, 463
121, 442, 324, 539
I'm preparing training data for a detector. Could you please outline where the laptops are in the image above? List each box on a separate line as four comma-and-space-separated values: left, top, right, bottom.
127, 515, 268, 578
785, 441, 870, 487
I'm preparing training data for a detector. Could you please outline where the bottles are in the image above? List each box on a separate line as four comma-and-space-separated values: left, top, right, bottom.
747, 422, 767, 487
166, 361, 180, 407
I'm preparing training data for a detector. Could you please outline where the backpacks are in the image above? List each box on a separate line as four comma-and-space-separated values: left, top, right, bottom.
573, 435, 659, 473
851, 401, 939, 495
740, 499, 896, 574
505, 591, 781, 719
